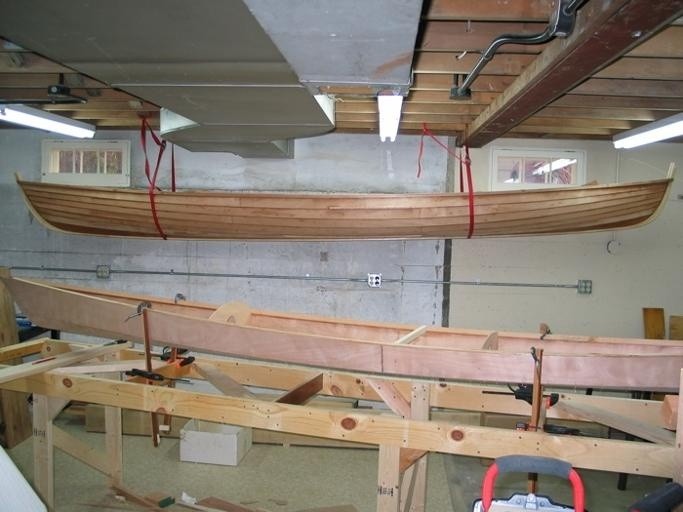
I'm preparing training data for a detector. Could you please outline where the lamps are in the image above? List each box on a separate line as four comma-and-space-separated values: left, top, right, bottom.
377, 89, 404, 143
0, 103, 97, 139
612, 112, 683, 149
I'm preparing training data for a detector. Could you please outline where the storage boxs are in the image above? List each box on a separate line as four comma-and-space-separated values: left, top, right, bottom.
179, 419, 253, 466
